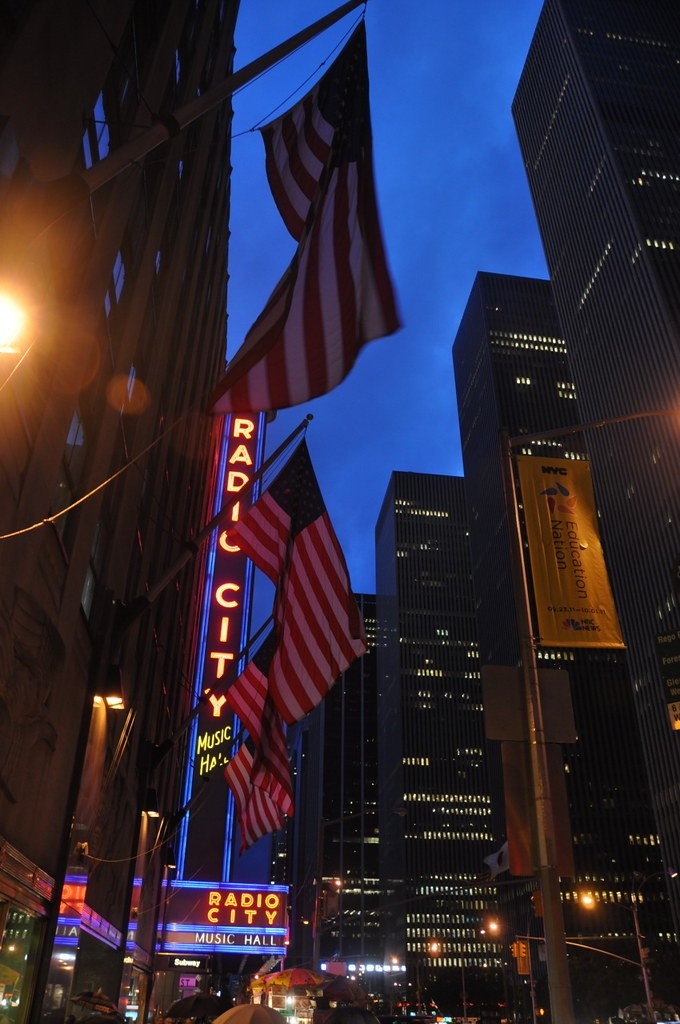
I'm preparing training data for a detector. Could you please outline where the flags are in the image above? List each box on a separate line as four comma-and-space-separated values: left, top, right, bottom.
223, 734, 286, 858
227, 438, 368, 725
226, 627, 294, 816
205, 17, 400, 413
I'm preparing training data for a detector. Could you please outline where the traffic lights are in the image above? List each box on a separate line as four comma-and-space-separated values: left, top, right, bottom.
510, 943, 518, 958
519, 942, 526, 957
536, 1008, 544, 1016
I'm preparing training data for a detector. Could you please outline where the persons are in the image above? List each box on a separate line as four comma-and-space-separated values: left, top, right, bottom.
65, 1016, 76, 1024
154, 1015, 212, 1024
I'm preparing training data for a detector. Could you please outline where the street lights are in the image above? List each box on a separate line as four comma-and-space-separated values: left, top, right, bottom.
491, 922, 538, 1024
584, 867, 680, 1024
431, 944, 468, 1024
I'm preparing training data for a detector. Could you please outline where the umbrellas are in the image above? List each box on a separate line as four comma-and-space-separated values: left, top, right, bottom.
248, 967, 328, 990
211, 1003, 287, 1024
163, 992, 233, 1016
324, 1006, 381, 1024
68, 991, 119, 1012
326, 975, 367, 1000
78, 1011, 120, 1024
